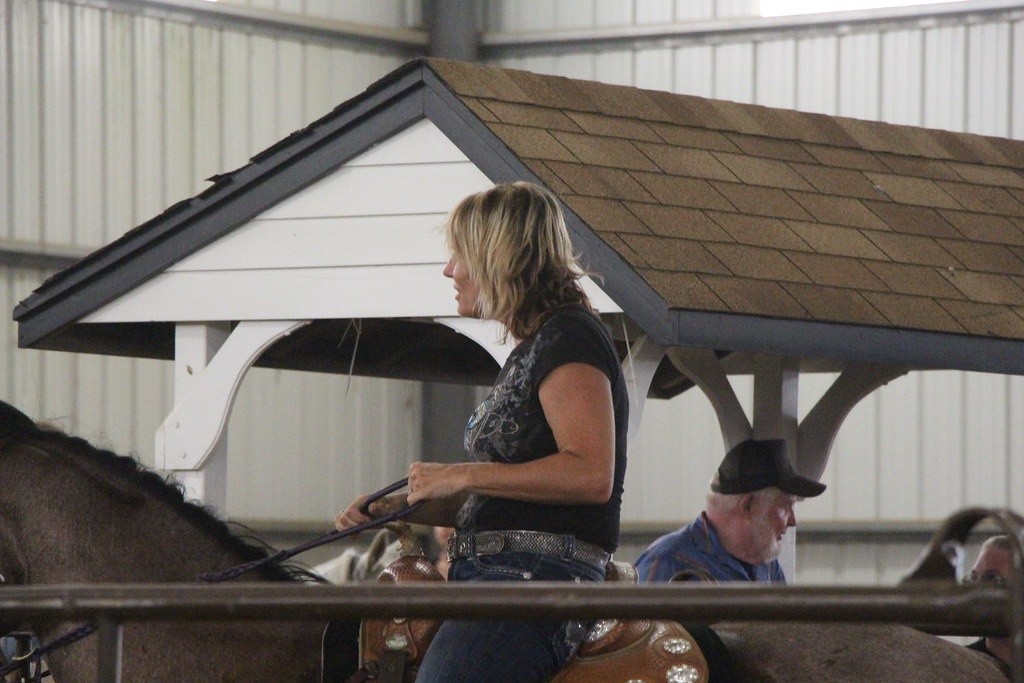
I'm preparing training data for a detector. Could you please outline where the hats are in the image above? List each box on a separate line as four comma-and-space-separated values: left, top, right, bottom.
711, 438, 826, 498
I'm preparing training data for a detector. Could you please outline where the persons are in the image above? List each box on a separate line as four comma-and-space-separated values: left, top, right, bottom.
333, 182, 630, 683
958, 533, 1023, 683
635, 437, 828, 587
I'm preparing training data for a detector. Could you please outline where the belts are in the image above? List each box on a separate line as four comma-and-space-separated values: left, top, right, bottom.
445, 530, 610, 574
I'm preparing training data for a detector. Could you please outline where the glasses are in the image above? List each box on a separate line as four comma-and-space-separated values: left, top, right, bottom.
962, 574, 1008, 588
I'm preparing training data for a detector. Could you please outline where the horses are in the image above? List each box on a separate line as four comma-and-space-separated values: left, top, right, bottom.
0, 392, 1016, 683
